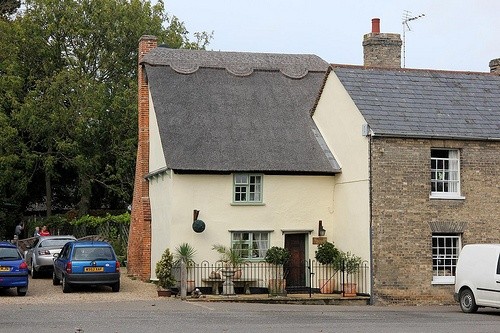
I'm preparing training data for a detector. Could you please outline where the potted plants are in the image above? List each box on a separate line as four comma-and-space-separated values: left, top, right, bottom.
155, 247, 177, 297
171, 242, 199, 292
314, 240, 340, 294
332, 250, 369, 297
211, 236, 259, 279
264, 246, 292, 294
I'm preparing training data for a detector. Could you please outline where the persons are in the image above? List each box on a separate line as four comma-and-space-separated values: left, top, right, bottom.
34, 226, 41, 237
39, 225, 51, 237
15, 219, 25, 240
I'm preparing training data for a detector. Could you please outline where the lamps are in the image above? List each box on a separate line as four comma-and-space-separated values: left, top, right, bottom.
319, 226, 326, 237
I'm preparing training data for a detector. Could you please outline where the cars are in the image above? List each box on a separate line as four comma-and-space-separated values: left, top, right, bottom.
0, 241, 29, 296
52, 241, 120, 293
25, 235, 83, 280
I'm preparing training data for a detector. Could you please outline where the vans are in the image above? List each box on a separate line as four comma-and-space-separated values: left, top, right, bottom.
453, 243, 499, 314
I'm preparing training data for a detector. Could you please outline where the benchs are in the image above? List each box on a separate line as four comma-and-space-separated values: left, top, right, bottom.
201, 277, 264, 295
